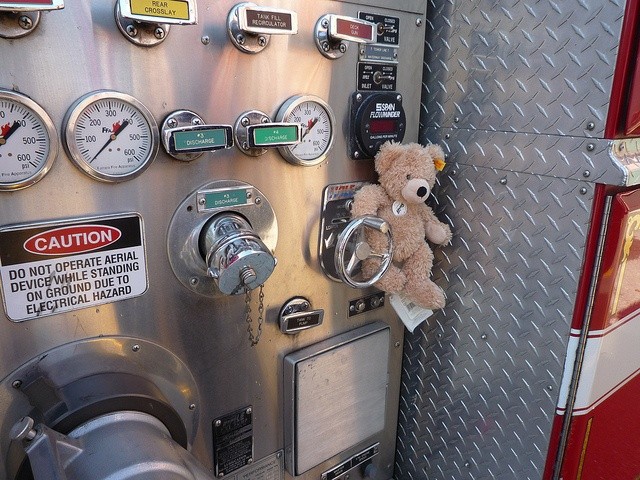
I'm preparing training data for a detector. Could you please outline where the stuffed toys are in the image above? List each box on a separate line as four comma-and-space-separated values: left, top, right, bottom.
351, 139, 452, 310
